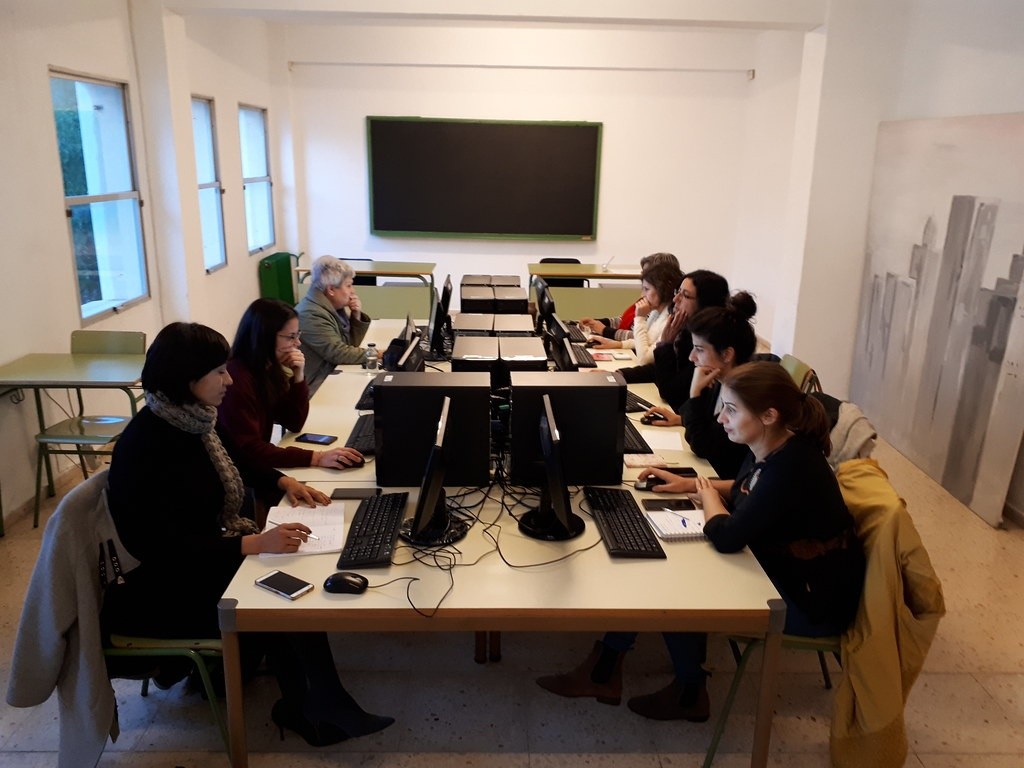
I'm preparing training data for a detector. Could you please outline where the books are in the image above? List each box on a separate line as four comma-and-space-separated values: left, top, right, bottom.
592, 353, 614, 361
611, 352, 632, 360
647, 509, 704, 539
623, 454, 667, 468
258, 502, 344, 558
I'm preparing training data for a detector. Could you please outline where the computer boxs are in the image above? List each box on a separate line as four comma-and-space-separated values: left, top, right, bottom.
508, 370, 624, 485
451, 275, 548, 453
371, 371, 490, 488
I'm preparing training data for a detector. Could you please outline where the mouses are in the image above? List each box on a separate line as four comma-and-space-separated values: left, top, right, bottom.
641, 415, 662, 425
634, 476, 666, 490
336, 456, 365, 469
323, 572, 369, 594
562, 320, 577, 326
584, 342, 600, 348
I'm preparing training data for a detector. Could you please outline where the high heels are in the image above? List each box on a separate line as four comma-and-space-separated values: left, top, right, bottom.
307, 684, 395, 738
271, 696, 351, 747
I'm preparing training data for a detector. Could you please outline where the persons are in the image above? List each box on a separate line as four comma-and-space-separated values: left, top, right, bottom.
211, 298, 363, 470
536, 361, 866, 722
578, 253, 688, 367
294, 255, 388, 400
591, 269, 729, 414
644, 292, 783, 479
106, 323, 395, 749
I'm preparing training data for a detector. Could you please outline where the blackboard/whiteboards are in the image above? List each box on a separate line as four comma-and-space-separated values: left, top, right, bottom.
365, 115, 602, 242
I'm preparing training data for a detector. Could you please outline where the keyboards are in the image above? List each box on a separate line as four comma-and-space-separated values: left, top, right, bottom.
582, 486, 667, 559
344, 413, 376, 455
355, 379, 375, 411
566, 325, 587, 343
336, 491, 410, 570
398, 325, 428, 341
624, 414, 654, 454
572, 344, 597, 368
626, 389, 656, 413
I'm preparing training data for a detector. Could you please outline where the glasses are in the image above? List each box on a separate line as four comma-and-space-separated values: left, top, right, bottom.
278, 330, 302, 343
674, 289, 697, 301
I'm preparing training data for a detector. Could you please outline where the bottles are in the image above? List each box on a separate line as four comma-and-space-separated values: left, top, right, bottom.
365, 343, 379, 379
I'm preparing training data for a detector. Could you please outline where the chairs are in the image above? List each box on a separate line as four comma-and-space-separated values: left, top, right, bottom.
33, 331, 147, 529
692, 352, 946, 768
6, 468, 224, 768
338, 258, 376, 286
540, 258, 589, 288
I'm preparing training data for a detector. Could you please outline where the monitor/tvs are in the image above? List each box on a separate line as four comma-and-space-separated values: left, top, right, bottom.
517, 394, 586, 542
398, 274, 456, 372
398, 396, 468, 547
533, 274, 579, 372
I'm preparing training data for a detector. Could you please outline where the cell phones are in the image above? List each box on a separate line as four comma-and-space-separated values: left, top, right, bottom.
641, 499, 696, 510
295, 433, 338, 445
331, 488, 382, 500
255, 570, 314, 600
656, 467, 698, 477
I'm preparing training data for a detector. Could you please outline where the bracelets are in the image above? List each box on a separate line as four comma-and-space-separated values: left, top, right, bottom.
318, 452, 322, 467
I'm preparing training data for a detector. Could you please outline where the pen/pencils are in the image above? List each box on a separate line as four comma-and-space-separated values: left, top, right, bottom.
661, 507, 700, 525
637, 402, 665, 418
269, 520, 320, 539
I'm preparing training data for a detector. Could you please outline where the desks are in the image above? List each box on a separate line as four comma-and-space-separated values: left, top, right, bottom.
0, 353, 146, 536
294, 261, 436, 312
217, 317, 786, 768
528, 263, 643, 287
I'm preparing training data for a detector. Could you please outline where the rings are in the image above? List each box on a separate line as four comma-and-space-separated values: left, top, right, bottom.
698, 487, 702, 490
336, 455, 338, 461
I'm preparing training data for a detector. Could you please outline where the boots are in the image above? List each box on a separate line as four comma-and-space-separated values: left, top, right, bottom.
627, 671, 712, 722
536, 640, 634, 705
